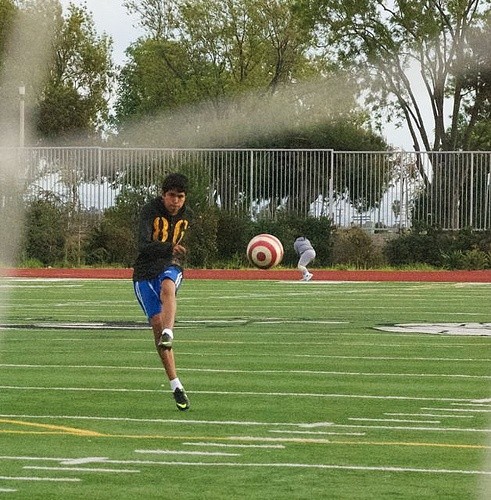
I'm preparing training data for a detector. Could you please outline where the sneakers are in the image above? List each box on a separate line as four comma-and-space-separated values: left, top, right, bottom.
172, 387, 190, 412
158, 334, 174, 349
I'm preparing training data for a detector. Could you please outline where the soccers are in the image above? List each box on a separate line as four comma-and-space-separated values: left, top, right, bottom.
246, 233, 285, 270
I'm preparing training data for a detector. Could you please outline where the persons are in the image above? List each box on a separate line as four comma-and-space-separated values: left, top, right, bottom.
294, 233, 316, 281
132, 171, 194, 410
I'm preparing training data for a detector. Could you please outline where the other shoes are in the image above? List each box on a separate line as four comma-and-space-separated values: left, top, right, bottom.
300, 276, 306, 281
306, 273, 313, 281
